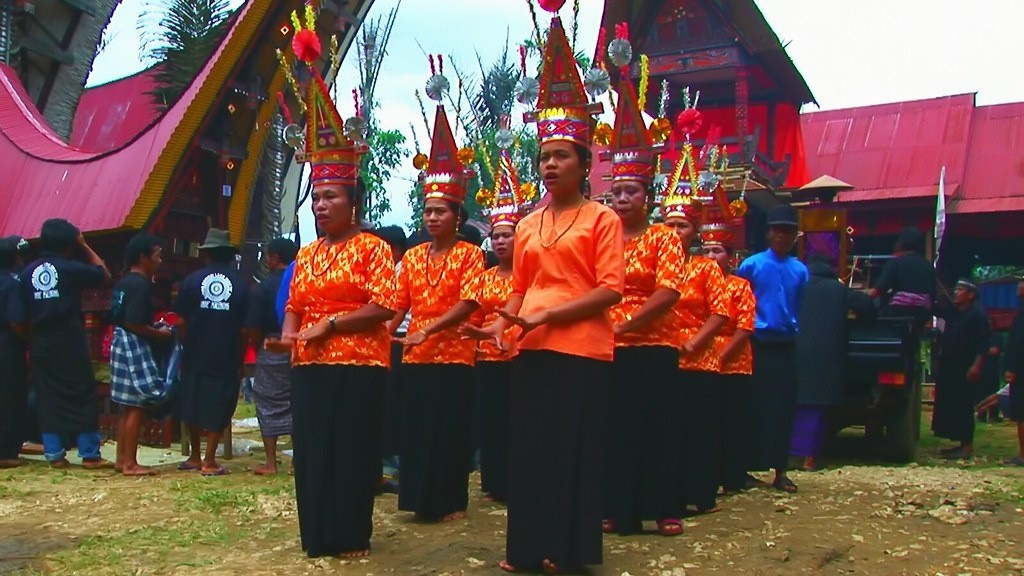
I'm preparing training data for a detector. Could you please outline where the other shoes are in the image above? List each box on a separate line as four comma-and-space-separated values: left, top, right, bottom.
773, 472, 800, 492
657, 517, 684, 536
939, 445, 968, 460
742, 476, 765, 488
999, 456, 1023, 467
601, 519, 618, 533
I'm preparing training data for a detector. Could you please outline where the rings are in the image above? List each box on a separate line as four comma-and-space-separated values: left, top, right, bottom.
406, 339, 409, 342
302, 333, 306, 338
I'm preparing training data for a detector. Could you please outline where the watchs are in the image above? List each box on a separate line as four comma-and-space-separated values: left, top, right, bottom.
328, 314, 336, 331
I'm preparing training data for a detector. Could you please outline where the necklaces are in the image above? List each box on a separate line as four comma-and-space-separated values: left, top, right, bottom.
627, 225, 648, 262
425, 242, 455, 287
311, 236, 352, 277
491, 266, 505, 294
537, 194, 585, 249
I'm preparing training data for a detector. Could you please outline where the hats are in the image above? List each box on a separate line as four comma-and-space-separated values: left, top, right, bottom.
696, 146, 751, 248
515, 0, 604, 150
765, 203, 800, 225
657, 83, 722, 234
954, 276, 978, 293
474, 115, 535, 227
275, 5, 369, 185
196, 227, 239, 251
406, 52, 476, 205
586, 20, 673, 182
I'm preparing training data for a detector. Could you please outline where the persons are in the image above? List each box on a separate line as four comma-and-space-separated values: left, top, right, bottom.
172, 227, 251, 476
17, 218, 116, 470
599, 149, 685, 543
498, 125, 625, 572
0, 234, 38, 469
264, 165, 397, 569
390, 181, 486, 528
241, 201, 1024, 515
109, 232, 174, 476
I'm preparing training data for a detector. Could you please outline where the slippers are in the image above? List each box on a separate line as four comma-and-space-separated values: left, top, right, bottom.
177, 461, 199, 470
201, 464, 230, 475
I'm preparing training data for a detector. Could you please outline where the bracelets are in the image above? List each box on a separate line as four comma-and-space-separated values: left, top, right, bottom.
419, 329, 427, 335
625, 312, 633, 322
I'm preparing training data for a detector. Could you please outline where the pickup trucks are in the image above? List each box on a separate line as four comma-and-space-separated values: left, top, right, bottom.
824, 255, 938, 464
